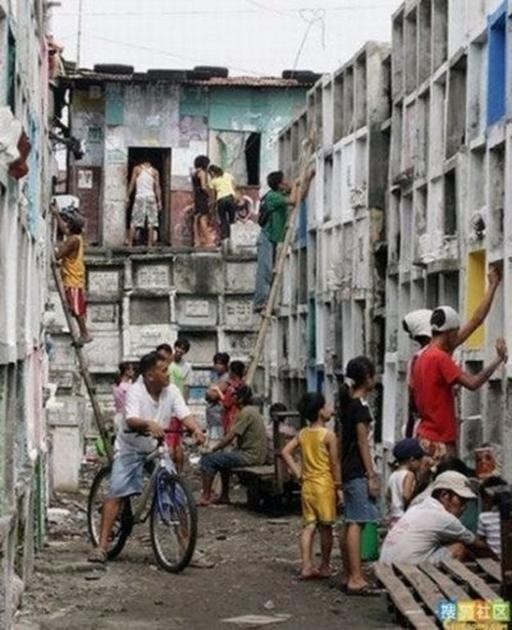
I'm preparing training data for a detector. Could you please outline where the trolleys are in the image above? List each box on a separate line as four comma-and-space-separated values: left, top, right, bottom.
231, 404, 302, 516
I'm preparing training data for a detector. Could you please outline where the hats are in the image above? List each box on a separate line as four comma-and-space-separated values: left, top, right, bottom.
393, 438, 420, 461
433, 470, 477, 498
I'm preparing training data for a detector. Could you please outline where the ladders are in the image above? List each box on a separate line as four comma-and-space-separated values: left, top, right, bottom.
49, 242, 115, 467
245, 143, 314, 387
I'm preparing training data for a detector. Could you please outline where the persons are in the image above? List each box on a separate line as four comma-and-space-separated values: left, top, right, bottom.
124, 160, 163, 254
90, 265, 508, 610
254, 171, 314, 309
49, 198, 93, 344
192, 154, 255, 247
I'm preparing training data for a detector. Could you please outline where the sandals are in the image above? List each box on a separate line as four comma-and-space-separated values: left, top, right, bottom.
178, 550, 216, 568
87, 548, 109, 564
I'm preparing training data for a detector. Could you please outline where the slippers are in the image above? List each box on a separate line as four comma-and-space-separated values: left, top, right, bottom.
346, 583, 384, 596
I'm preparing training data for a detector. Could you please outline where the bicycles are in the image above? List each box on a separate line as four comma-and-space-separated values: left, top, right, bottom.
85, 429, 211, 575
